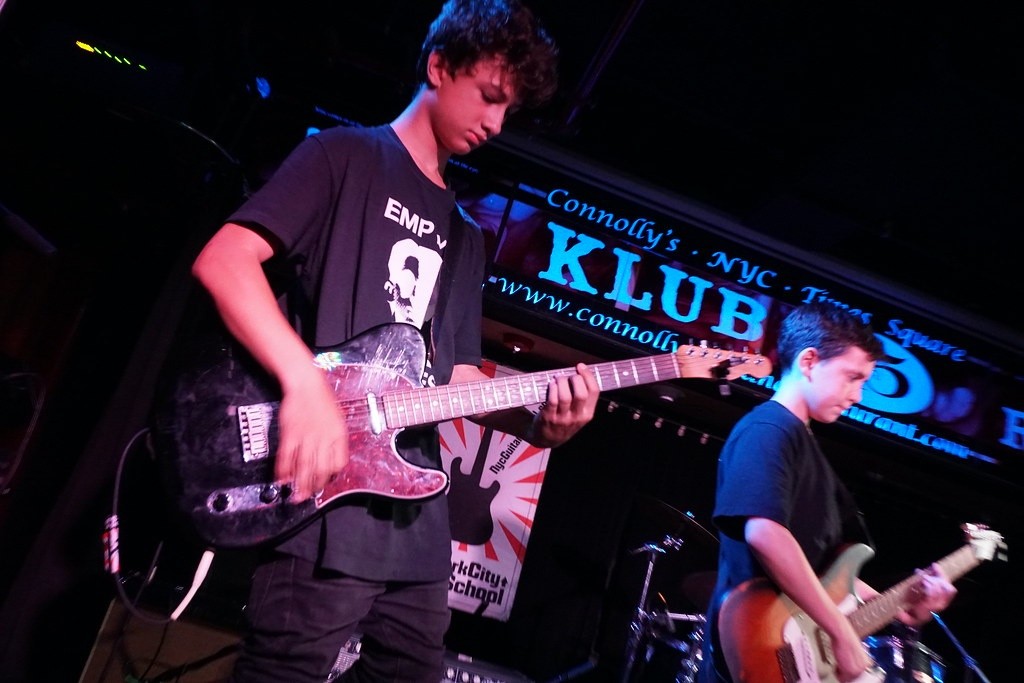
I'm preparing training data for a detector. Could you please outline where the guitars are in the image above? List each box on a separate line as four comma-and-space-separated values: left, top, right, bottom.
710, 522, 1007, 683
167, 322, 773, 547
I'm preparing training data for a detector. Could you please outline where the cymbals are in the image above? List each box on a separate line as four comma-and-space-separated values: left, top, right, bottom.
638, 492, 723, 547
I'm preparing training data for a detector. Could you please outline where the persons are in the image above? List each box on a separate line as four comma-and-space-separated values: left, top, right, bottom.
707, 305, 955, 683
193, 0, 599, 683
